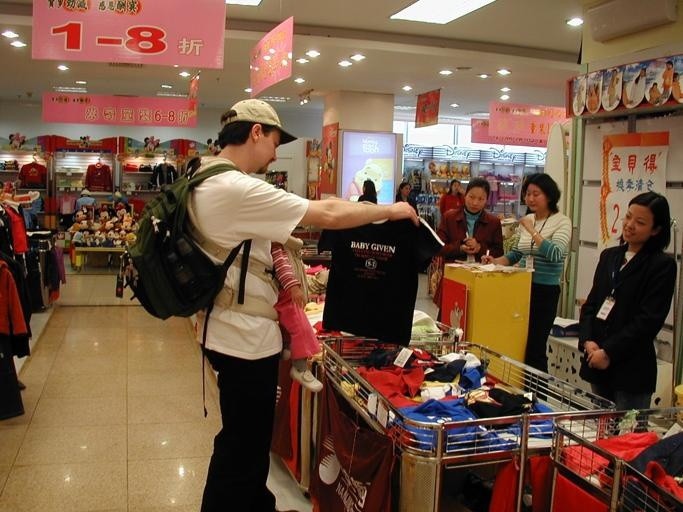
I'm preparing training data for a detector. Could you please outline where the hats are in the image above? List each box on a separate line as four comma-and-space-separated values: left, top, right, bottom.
220, 98, 297, 144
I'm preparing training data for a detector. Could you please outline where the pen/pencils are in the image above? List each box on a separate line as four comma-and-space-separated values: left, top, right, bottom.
486, 249, 490, 264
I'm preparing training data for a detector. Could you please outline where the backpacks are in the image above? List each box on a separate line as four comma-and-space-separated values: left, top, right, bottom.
115, 155, 251, 320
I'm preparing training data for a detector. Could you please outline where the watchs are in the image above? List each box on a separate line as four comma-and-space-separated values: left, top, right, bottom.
532, 232, 539, 238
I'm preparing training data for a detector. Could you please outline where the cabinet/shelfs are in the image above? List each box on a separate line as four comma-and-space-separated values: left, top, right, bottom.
308, 340, 615, 512
548, 408, 683, 512
401, 143, 547, 239
0, 148, 201, 231
74, 246, 125, 274
270, 297, 459, 499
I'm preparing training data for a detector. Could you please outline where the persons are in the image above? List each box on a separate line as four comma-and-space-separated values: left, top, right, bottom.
485, 172, 498, 206
479, 174, 574, 395
266, 228, 328, 393
439, 180, 465, 218
431, 179, 509, 325
357, 180, 378, 204
576, 193, 678, 434
395, 183, 423, 212
181, 101, 419, 511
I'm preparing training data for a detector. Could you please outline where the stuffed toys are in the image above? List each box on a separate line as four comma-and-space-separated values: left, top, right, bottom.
73, 209, 141, 249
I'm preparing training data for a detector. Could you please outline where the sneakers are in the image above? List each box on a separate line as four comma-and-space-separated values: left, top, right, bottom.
282, 349, 314, 362
289, 365, 324, 392
